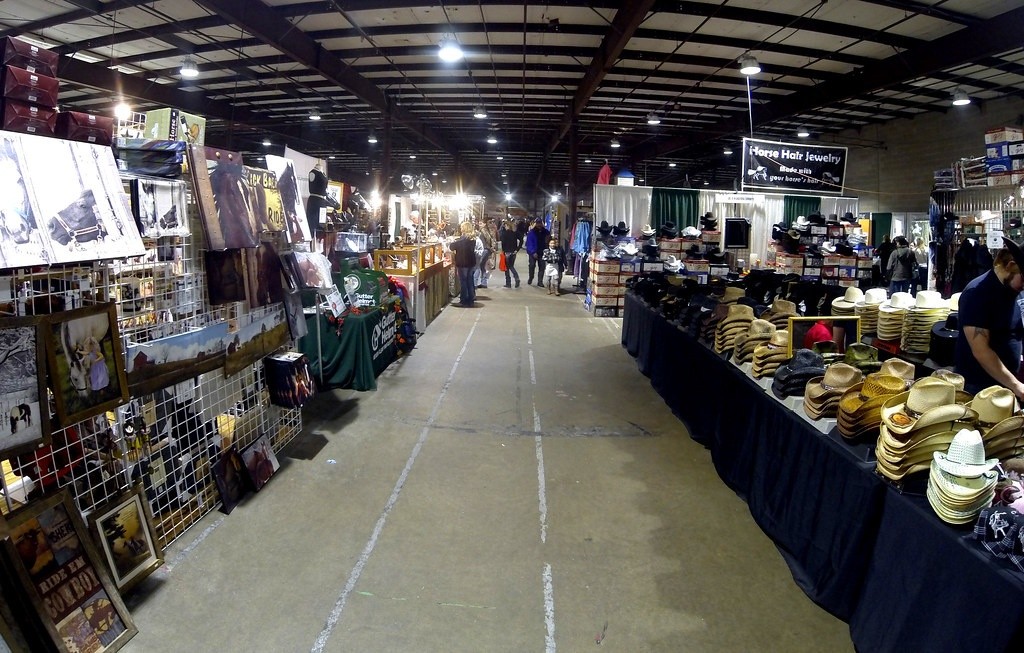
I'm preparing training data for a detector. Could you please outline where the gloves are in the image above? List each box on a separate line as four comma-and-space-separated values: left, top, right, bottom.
533, 253, 538, 259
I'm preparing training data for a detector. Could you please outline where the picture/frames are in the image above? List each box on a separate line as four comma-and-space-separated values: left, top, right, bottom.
0, 301, 130, 461
0, 482, 165, 653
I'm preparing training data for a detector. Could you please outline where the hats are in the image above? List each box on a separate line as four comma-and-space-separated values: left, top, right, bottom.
602, 238, 638, 257
596, 221, 630, 235
1001, 236, 1024, 274
686, 245, 704, 259
700, 212, 717, 227
654, 272, 1024, 524
709, 245, 725, 263
535, 217, 542, 222
681, 226, 702, 237
664, 255, 681, 273
1009, 216, 1022, 227
642, 238, 660, 256
943, 212, 959, 221
895, 236, 905, 241
772, 213, 875, 256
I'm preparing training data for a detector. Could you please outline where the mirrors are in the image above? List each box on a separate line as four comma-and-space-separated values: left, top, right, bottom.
787, 316, 861, 360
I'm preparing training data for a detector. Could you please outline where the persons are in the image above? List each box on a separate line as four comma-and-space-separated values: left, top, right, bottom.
512, 218, 534, 247
875, 235, 929, 299
554, 238, 567, 292
805, 320, 832, 349
500, 222, 523, 289
449, 222, 476, 307
955, 237, 1024, 401
405, 210, 422, 241
525, 217, 552, 288
76, 337, 110, 392
542, 240, 563, 296
832, 318, 857, 354
473, 214, 503, 288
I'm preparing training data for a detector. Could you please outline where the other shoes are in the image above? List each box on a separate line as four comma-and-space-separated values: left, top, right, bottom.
504, 284, 511, 288
528, 275, 534, 284
515, 280, 520, 287
451, 303, 474, 307
477, 284, 487, 288
538, 283, 544, 287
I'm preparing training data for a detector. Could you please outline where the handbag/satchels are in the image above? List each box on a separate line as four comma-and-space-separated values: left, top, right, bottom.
912, 269, 919, 279
499, 251, 507, 271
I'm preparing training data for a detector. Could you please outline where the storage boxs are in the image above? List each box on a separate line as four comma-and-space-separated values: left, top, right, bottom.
0, 35, 206, 178
984, 127, 1024, 187
765, 225, 874, 288
584, 230, 729, 318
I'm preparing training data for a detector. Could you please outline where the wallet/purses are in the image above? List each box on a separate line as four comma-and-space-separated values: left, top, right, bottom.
640, 221, 679, 239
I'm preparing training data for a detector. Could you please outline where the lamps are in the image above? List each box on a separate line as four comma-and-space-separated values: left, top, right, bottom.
367, 132, 377, 143
610, 139, 620, 148
180, 60, 200, 76
647, 114, 661, 125
486, 135, 498, 144
952, 88, 971, 106
262, 138, 271, 146
739, 51, 761, 75
474, 108, 487, 119
724, 147, 733, 155
439, 38, 463, 63
797, 127, 810, 138
309, 108, 321, 120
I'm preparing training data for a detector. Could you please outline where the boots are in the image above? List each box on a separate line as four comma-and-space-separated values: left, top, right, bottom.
555, 283, 560, 296
546, 282, 551, 295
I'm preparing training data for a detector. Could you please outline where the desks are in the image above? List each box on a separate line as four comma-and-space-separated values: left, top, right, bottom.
621, 291, 1024, 653
396, 262, 453, 335
297, 294, 400, 392
